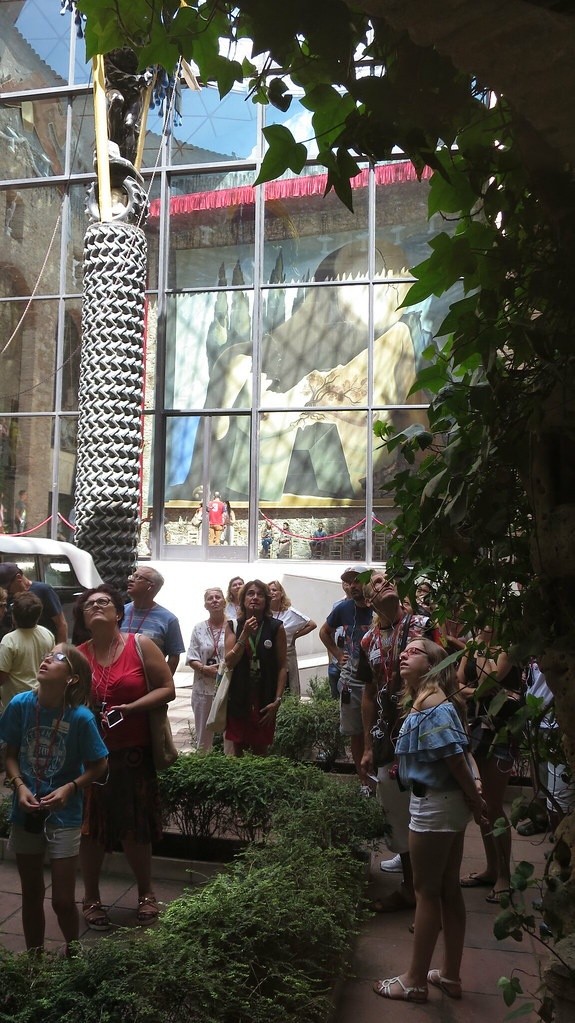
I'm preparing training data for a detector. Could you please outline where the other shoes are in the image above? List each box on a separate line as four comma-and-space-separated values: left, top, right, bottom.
361, 785, 373, 798
516, 816, 549, 835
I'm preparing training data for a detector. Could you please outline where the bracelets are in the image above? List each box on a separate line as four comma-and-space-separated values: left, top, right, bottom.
17, 783, 25, 790
72, 780, 78, 791
11, 775, 21, 782
475, 777, 482, 782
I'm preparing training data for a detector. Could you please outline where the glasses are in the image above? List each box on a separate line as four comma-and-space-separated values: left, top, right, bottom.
82, 596, 116, 611
41, 650, 74, 676
128, 573, 153, 583
399, 647, 428, 660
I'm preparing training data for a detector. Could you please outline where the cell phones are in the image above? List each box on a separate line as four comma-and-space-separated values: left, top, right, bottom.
106, 710, 124, 729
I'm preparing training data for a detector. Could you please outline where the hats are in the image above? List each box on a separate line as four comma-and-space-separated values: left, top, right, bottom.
0, 562, 20, 589
341, 567, 368, 583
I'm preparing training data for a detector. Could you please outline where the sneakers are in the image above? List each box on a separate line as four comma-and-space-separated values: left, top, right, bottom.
380, 852, 404, 873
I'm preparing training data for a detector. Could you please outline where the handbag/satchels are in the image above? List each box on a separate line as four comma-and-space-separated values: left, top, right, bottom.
204, 658, 235, 733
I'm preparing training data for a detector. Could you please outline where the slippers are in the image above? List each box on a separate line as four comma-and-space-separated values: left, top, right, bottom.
371, 891, 416, 912
486, 882, 512, 903
459, 871, 496, 887
409, 920, 416, 932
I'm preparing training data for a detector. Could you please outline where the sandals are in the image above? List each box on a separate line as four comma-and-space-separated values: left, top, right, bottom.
427, 968, 462, 998
81, 897, 110, 931
373, 974, 426, 1003
136, 893, 158, 926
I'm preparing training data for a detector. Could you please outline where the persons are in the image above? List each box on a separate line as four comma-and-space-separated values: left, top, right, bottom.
350, 526, 398, 560
323, 564, 575, 929
58, 506, 76, 543
185, 575, 317, 757
276, 522, 292, 558
73, 585, 176, 928
1, 642, 109, 963
0, 490, 26, 536
373, 637, 488, 998
310, 523, 338, 560
196, 492, 235, 546
0, 559, 184, 767
261, 520, 274, 556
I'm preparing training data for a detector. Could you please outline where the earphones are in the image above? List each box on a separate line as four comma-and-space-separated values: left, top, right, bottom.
67, 678, 73, 683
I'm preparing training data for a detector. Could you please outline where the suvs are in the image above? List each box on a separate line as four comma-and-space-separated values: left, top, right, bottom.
0, 535, 111, 643
